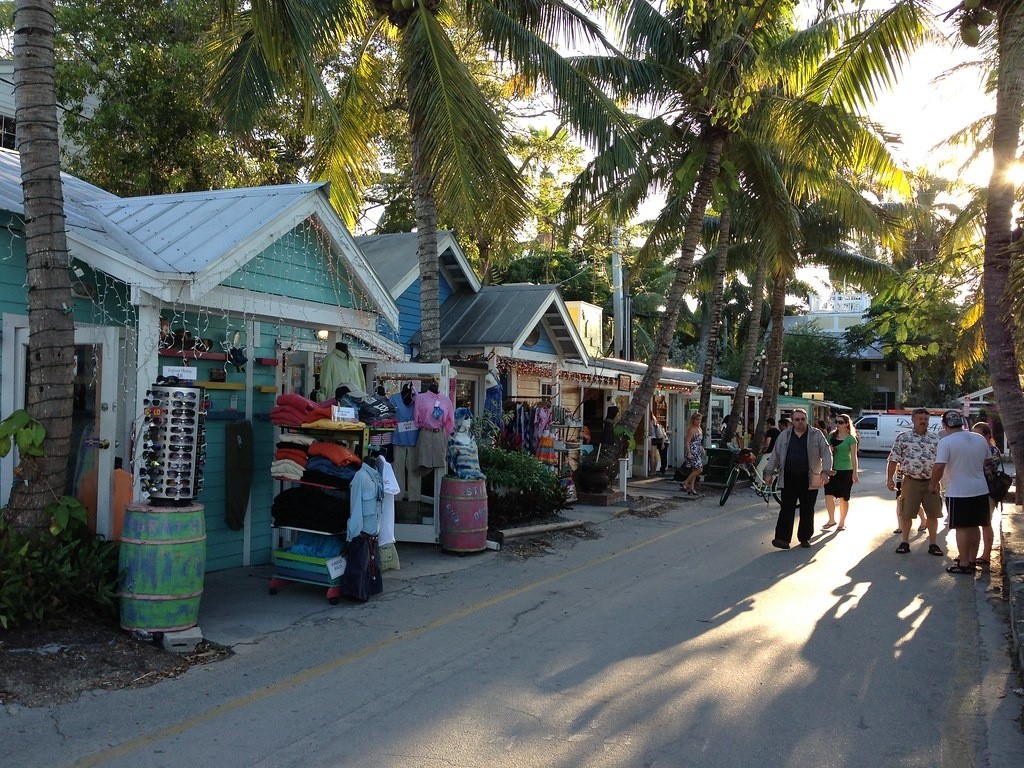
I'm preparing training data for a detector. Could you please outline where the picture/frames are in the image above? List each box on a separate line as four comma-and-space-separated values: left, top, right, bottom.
618, 374, 632, 392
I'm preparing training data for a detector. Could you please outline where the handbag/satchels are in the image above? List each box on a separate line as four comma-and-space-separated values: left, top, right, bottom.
655, 422, 663, 438
987, 470, 1013, 501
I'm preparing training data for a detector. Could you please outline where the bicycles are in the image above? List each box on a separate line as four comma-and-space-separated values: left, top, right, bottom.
719, 447, 802, 509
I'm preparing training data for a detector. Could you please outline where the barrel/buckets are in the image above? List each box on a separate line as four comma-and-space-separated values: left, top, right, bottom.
438, 474, 489, 553
119, 500, 208, 632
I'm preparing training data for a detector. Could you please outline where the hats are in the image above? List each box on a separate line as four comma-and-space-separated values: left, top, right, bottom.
943, 410, 963, 426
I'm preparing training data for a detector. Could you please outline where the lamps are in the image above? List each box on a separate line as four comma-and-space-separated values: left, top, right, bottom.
315, 330, 329, 341
70, 281, 95, 299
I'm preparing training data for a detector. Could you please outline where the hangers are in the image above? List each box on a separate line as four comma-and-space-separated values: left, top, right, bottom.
503, 395, 550, 409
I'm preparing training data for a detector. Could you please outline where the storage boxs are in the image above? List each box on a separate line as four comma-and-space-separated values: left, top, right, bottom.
273, 549, 342, 583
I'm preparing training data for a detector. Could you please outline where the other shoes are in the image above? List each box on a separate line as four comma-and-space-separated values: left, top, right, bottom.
648, 472, 656, 476
750, 485, 761, 489
772, 539, 790, 549
801, 540, 810, 547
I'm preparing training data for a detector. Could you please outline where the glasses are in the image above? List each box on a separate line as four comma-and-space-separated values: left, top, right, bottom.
834, 420, 847, 425
792, 418, 806, 422
139, 376, 212, 498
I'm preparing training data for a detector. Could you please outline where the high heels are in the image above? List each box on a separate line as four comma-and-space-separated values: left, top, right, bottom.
688, 489, 699, 495
679, 482, 688, 491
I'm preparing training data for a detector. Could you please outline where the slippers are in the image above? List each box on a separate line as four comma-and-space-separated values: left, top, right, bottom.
928, 544, 944, 556
954, 555, 961, 561
975, 557, 990, 565
838, 525, 846, 530
894, 529, 903, 533
918, 519, 928, 530
823, 521, 837, 529
896, 542, 910, 553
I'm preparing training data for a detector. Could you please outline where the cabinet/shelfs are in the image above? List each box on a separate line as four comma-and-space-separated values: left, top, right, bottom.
656, 404, 666, 421
268, 423, 397, 606
158, 348, 245, 419
551, 424, 583, 477
253, 358, 279, 421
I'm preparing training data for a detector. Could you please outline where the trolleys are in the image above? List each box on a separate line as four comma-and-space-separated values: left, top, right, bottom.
268, 424, 397, 605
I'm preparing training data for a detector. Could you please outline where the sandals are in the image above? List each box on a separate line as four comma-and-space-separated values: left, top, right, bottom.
945, 561, 977, 574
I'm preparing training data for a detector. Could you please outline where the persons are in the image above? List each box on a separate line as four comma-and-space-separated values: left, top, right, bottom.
657, 423, 670, 474
885, 408, 1002, 575
538, 430, 556, 463
680, 412, 704, 495
649, 414, 659, 476
718, 408, 860, 550
319, 344, 502, 541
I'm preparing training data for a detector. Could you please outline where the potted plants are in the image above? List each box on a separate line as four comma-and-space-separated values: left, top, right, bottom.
579, 455, 613, 494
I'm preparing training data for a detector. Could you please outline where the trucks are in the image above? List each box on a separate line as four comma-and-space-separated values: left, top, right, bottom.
852, 415, 969, 452
